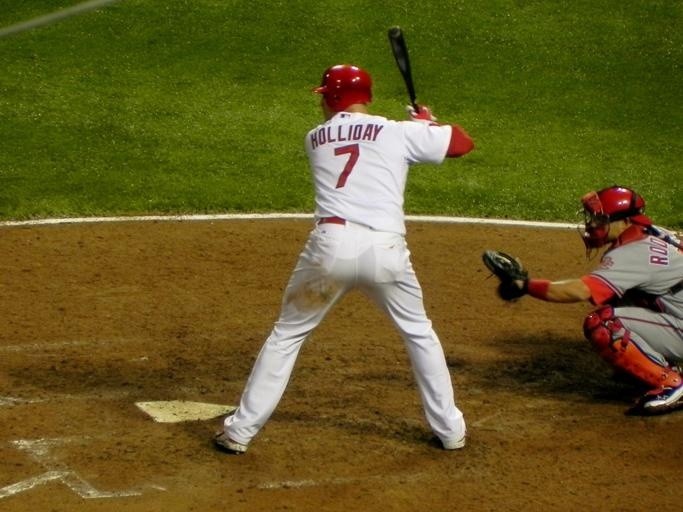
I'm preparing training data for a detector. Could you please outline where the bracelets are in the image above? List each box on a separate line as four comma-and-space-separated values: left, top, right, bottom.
528, 280, 549, 300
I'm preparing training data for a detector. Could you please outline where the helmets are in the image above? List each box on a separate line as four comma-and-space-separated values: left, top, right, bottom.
576, 184, 652, 261
311, 64, 373, 112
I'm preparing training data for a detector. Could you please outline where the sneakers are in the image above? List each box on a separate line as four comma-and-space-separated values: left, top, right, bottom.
216, 431, 248, 452
442, 436, 466, 449
644, 383, 683, 408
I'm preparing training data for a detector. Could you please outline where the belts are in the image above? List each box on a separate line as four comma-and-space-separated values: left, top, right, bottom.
318, 216, 346, 225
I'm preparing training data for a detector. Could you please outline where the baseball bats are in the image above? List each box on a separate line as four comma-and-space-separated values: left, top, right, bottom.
388, 25, 418, 112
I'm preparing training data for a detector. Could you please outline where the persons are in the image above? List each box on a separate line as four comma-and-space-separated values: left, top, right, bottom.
482, 185, 683, 414
212, 64, 475, 455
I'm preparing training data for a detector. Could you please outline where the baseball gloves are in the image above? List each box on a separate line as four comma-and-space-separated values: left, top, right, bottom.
482, 249, 528, 300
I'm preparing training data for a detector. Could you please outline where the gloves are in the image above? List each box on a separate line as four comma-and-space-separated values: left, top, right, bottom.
407, 104, 439, 126
